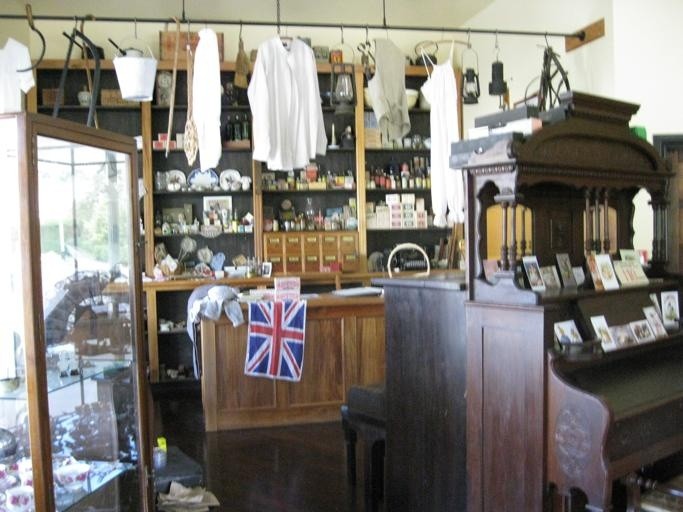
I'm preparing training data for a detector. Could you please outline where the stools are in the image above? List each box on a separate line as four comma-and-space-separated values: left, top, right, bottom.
339, 383, 384, 509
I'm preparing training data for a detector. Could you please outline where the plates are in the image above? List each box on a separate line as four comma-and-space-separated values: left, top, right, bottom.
165, 167, 242, 191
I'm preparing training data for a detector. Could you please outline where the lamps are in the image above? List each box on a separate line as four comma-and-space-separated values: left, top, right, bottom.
330, 27, 360, 117
459, 28, 480, 103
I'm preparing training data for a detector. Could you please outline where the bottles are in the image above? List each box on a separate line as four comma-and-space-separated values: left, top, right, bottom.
305, 197, 314, 232
154, 170, 166, 191
232, 209, 241, 232
365, 155, 431, 191
225, 112, 249, 141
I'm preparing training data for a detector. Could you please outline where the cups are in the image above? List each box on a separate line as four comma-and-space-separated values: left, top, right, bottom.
245, 255, 259, 280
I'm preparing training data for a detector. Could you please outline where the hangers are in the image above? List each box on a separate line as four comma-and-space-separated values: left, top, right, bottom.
194, 21, 213, 36
260, 21, 312, 58
358, 26, 414, 66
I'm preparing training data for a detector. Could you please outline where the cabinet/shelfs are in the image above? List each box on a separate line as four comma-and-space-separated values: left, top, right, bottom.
448, 89, 683, 511
36, 61, 462, 388
2, 113, 151, 512
196, 293, 388, 431
372, 275, 471, 511
